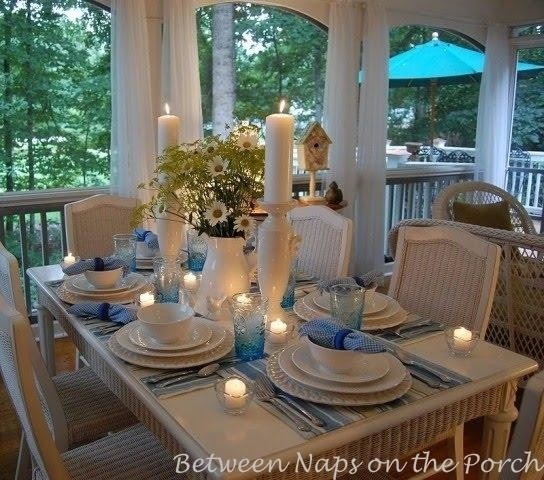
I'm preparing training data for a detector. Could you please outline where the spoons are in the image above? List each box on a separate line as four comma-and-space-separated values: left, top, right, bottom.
389, 349, 450, 390
159, 364, 220, 387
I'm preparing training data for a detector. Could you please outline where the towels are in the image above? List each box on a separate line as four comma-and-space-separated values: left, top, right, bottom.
318, 271, 386, 295
127, 223, 159, 249
65, 299, 140, 328
296, 318, 387, 354
182, 250, 206, 272
61, 255, 132, 277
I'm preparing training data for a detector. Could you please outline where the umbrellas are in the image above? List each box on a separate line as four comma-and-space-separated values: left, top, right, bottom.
358, 32, 544, 148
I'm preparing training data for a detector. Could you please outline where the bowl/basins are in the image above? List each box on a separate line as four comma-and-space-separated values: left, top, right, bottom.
333, 283, 379, 309
137, 303, 193, 344
307, 336, 363, 374
84, 268, 123, 289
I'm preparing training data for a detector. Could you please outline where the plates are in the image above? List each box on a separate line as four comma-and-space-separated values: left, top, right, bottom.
56, 274, 148, 306
292, 288, 406, 331
106, 318, 235, 368
265, 342, 412, 407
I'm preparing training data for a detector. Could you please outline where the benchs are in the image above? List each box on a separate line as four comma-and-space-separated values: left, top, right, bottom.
389, 216, 544, 390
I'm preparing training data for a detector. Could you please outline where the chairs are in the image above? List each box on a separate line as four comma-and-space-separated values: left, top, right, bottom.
284, 204, 354, 285
432, 180, 537, 235
408, 144, 532, 165
388, 225, 501, 480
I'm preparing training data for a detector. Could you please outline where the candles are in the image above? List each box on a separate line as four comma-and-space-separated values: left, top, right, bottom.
270, 318, 286, 343
225, 377, 245, 408
265, 98, 294, 203
454, 326, 473, 352
158, 101, 182, 203
184, 272, 197, 291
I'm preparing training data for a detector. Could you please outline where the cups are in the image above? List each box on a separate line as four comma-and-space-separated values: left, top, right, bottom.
112, 227, 208, 302
331, 284, 362, 331
58, 256, 80, 273
278, 258, 296, 311
444, 326, 479, 361
263, 323, 290, 350
231, 292, 269, 361
214, 379, 255, 416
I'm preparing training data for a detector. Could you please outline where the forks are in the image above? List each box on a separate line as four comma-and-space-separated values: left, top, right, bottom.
252, 375, 326, 430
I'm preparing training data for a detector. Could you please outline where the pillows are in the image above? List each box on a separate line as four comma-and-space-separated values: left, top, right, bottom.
453, 200, 525, 263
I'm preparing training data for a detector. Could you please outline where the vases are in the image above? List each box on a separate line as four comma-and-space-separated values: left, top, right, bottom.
199, 237, 251, 312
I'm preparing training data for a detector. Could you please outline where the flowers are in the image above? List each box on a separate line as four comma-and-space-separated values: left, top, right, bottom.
131, 119, 266, 237
